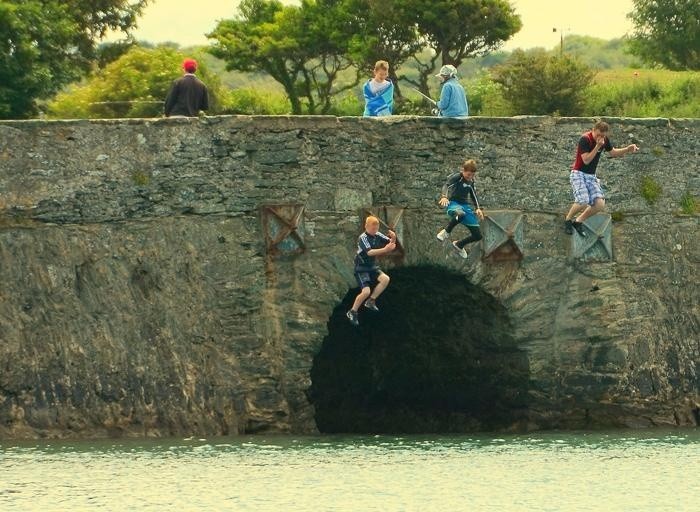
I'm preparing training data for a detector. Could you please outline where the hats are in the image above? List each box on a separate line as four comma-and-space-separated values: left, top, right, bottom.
182, 59, 199, 72
436, 64, 456, 79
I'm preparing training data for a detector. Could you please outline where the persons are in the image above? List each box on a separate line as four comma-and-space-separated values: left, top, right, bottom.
163, 58, 209, 118
564, 121, 641, 238
435, 158, 484, 259
431, 63, 470, 117
361, 60, 394, 116
345, 215, 397, 326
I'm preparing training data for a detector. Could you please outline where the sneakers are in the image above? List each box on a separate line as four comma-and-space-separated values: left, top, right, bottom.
346, 309, 359, 328
573, 220, 588, 238
437, 229, 451, 241
565, 218, 573, 235
364, 296, 379, 312
452, 241, 469, 259
